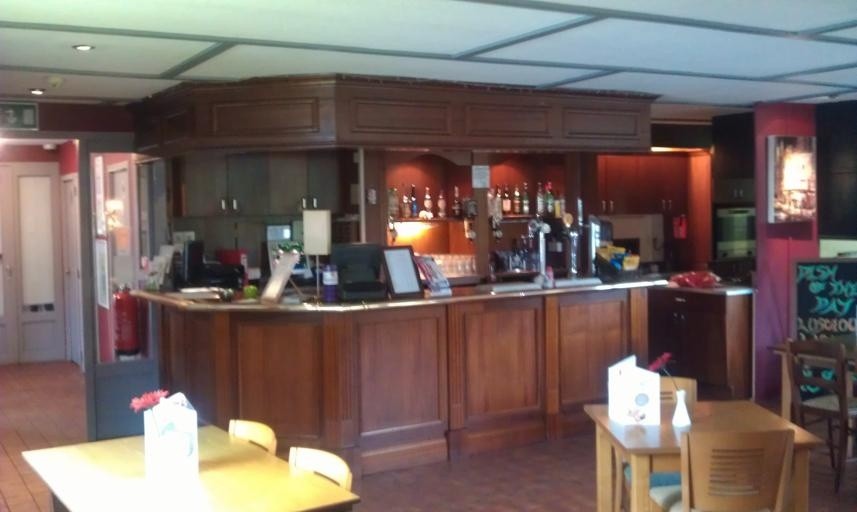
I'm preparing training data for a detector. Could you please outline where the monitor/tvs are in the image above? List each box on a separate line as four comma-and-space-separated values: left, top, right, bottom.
182, 238, 202, 284
329, 242, 383, 288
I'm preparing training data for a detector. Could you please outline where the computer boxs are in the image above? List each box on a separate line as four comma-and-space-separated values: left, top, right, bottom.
337, 281, 389, 303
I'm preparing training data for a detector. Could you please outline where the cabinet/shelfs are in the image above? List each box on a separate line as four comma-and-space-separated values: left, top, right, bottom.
360, 147, 714, 287
647, 287, 755, 400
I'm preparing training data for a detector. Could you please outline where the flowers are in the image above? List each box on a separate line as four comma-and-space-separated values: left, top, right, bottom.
648, 352, 682, 391
130, 388, 175, 414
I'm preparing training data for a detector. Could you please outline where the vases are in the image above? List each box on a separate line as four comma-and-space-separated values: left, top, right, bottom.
671, 390, 692, 428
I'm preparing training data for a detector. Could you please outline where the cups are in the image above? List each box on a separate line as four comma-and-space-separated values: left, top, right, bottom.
416, 253, 478, 279
242, 285, 258, 298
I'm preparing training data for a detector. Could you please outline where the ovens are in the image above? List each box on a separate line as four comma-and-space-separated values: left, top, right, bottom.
715, 207, 755, 260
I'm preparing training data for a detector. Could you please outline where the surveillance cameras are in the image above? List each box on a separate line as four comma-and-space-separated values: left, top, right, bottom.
43, 145, 57, 153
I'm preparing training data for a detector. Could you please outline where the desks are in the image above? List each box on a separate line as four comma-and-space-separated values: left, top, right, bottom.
21, 425, 361, 512
767, 338, 857, 420
583, 399, 826, 512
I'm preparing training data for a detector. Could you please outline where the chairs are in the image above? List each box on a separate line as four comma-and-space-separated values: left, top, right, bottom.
229, 418, 278, 454
784, 338, 853, 491
623, 376, 698, 490
649, 430, 794, 511
289, 447, 353, 490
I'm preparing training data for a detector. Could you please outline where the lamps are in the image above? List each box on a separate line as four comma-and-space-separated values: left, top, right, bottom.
105, 198, 126, 233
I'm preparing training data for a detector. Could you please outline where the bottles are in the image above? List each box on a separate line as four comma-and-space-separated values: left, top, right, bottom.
321, 263, 340, 303
485, 180, 565, 219
493, 233, 542, 273
388, 183, 462, 222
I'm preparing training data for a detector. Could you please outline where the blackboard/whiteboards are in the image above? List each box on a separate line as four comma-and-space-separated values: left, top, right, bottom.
789, 257, 857, 402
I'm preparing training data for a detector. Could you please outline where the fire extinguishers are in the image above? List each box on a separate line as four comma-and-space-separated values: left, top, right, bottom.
111, 283, 139, 356
